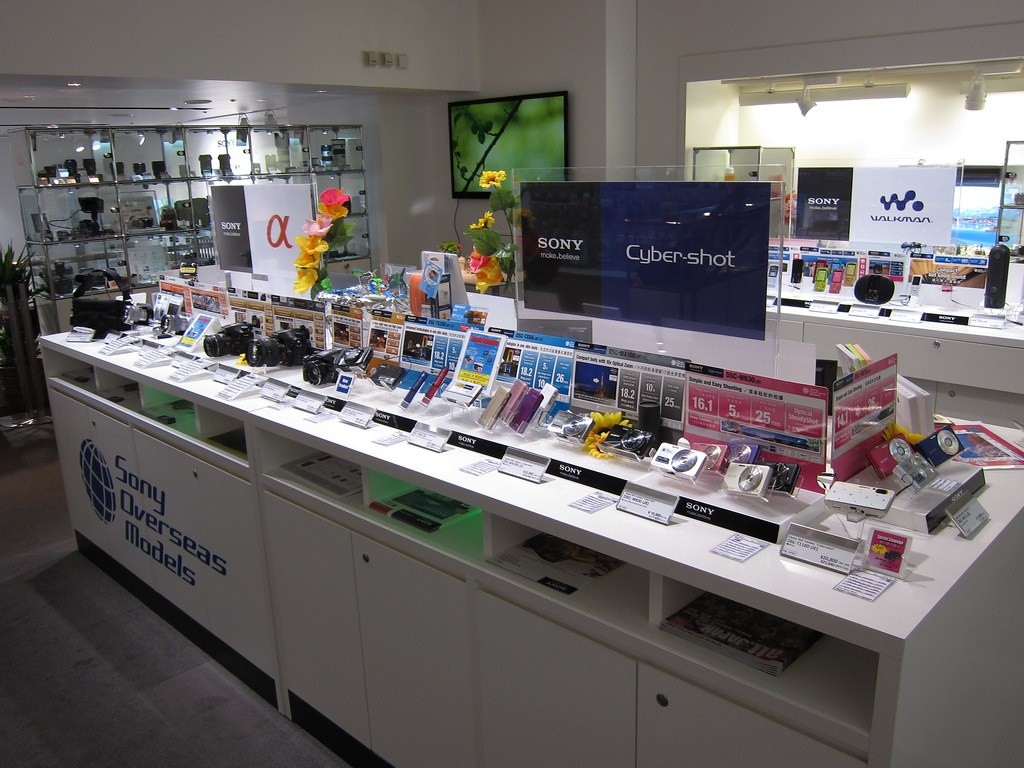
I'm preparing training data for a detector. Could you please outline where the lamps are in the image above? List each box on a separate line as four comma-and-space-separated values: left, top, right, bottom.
965, 63, 987, 110
795, 85, 817, 117
264, 109, 278, 125
739, 77, 911, 106
238, 114, 250, 125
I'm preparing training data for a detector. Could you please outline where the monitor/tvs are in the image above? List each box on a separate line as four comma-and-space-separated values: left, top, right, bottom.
447, 90, 568, 199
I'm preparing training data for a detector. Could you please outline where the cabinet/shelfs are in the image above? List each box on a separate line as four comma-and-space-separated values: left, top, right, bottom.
6, 124, 1024, 768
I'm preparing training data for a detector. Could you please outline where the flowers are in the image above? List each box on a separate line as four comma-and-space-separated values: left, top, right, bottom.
293, 188, 356, 293
464, 170, 534, 298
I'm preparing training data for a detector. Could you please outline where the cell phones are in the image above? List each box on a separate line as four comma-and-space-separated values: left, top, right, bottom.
768, 258, 857, 294
909, 275, 922, 295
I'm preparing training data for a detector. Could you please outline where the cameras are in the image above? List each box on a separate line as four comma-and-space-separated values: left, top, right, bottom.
122, 302, 374, 387
546, 409, 967, 518
